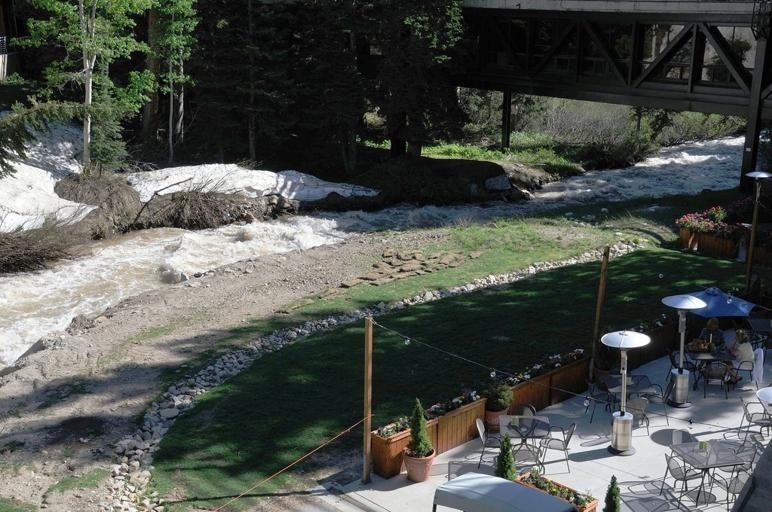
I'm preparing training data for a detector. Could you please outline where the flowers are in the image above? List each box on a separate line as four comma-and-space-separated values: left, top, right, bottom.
380, 390, 480, 438
675, 196, 772, 246
614, 314, 673, 331
521, 474, 594, 507
507, 349, 585, 386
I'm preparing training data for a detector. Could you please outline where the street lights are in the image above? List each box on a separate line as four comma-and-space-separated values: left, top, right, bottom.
744, 171, 772, 302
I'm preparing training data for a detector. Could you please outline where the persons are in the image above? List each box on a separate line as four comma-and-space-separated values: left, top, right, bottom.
718, 329, 755, 384
698, 318, 725, 349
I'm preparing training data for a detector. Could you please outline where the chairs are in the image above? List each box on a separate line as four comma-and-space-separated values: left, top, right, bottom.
513, 444, 542, 473
476, 418, 505, 470
536, 423, 576, 472
509, 404, 536, 452
585, 334, 772, 512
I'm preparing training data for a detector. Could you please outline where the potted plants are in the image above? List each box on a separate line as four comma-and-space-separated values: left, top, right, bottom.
403, 397, 435, 482
485, 381, 513, 431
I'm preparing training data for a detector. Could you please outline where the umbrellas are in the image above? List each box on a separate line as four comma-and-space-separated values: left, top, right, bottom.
686, 286, 756, 348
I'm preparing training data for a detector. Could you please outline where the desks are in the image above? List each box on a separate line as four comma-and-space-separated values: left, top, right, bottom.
500, 415, 550, 475
